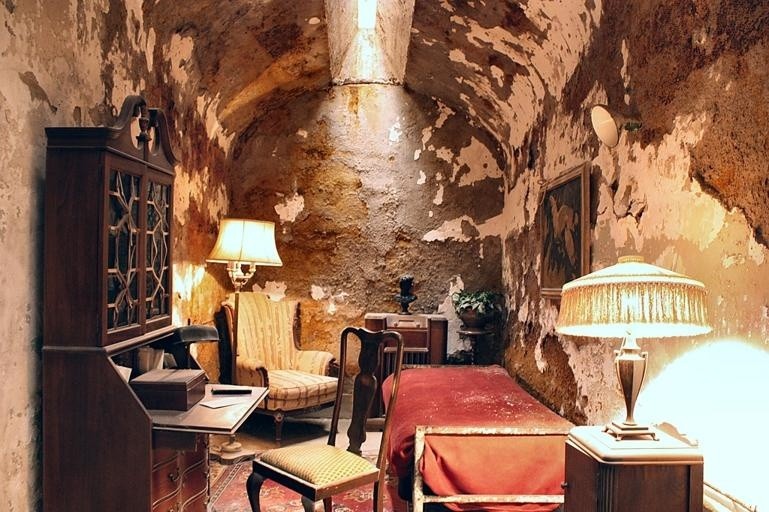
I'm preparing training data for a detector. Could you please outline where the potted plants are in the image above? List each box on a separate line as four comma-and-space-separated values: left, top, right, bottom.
451, 286, 502, 329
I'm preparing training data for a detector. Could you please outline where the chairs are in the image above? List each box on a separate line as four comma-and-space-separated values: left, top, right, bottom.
222, 289, 340, 445
241, 325, 406, 511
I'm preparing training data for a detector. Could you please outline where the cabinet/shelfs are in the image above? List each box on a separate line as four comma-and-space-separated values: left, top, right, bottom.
365, 314, 448, 417
36, 94, 270, 512
562, 423, 705, 512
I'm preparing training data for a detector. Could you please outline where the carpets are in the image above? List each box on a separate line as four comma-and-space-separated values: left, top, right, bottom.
206, 450, 411, 512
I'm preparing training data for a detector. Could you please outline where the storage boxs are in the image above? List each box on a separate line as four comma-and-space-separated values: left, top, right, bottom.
130, 367, 205, 412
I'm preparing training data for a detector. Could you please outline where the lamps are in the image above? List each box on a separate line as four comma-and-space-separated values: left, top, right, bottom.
203, 219, 283, 465
553, 253, 716, 442
168, 325, 219, 367
587, 104, 645, 147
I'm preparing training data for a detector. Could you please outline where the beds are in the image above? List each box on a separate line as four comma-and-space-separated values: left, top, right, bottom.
381, 362, 581, 511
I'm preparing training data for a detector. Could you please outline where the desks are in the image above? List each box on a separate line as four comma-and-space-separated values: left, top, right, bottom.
458, 330, 494, 365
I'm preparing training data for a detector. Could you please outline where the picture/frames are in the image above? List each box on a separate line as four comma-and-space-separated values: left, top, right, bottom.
535, 162, 594, 298
